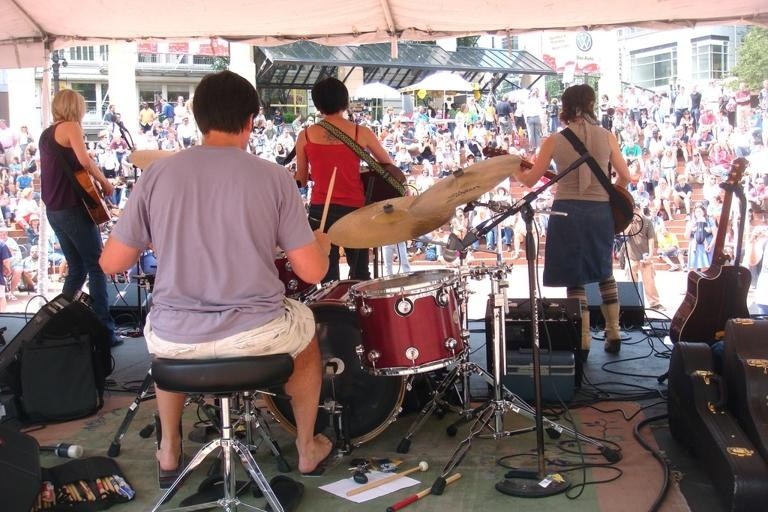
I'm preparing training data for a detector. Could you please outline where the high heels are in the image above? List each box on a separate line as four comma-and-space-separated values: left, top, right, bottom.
580, 334, 589, 363
602, 335, 622, 354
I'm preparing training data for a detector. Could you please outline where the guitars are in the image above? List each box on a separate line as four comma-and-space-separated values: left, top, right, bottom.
668, 156, 753, 350
73, 168, 112, 227
275, 155, 410, 206
482, 145, 637, 235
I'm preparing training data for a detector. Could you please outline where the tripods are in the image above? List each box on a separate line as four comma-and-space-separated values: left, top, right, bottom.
398, 251, 561, 453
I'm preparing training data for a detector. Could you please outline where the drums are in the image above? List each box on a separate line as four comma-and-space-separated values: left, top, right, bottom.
137, 248, 156, 293
351, 269, 469, 377
262, 279, 409, 450
273, 245, 318, 301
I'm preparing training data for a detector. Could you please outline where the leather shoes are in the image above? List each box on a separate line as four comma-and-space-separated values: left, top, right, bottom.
112, 334, 125, 348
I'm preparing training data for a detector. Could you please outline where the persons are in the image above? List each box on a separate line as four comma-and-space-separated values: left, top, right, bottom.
381, 95, 527, 278
608, 147, 768, 313
597, 81, 768, 146
82, 96, 155, 152
294, 114, 323, 134
295, 77, 391, 282
89, 153, 143, 248
514, 84, 631, 362
1, 118, 41, 300
154, 93, 203, 153
349, 112, 373, 131
98, 70, 333, 473
243, 126, 296, 164
39, 89, 124, 347
244, 106, 285, 139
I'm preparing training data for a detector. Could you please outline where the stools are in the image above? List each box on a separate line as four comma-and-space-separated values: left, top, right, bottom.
147, 353, 296, 512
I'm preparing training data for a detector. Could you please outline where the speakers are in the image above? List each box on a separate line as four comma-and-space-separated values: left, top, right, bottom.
0, 295, 116, 406
486, 298, 584, 392
97, 283, 147, 327
582, 280, 644, 328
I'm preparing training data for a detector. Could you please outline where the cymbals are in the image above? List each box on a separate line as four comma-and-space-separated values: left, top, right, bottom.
327, 195, 455, 249
129, 150, 176, 169
409, 154, 521, 214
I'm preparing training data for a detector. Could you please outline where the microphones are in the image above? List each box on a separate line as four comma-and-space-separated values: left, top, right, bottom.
111, 115, 128, 131
